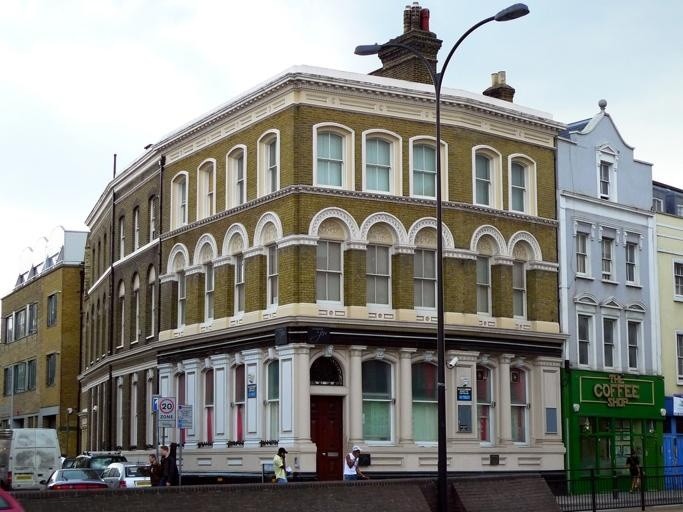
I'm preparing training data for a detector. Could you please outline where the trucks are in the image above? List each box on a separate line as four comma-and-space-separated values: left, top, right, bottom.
1, 428, 63, 490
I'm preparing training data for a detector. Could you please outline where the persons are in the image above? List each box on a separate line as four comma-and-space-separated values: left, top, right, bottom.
625, 451, 641, 493
144, 454, 162, 486
273, 447, 288, 483
160, 445, 179, 486
344, 446, 368, 480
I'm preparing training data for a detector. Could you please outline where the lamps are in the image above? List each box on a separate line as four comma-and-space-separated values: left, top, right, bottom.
579, 417, 590, 430
648, 421, 654, 434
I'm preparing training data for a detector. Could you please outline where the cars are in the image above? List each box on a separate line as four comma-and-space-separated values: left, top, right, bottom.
41, 467, 107, 489
0, 491, 27, 512
100, 461, 152, 487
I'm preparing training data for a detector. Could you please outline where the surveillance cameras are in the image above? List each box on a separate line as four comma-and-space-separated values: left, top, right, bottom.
448, 357, 458, 368
92, 405, 97, 412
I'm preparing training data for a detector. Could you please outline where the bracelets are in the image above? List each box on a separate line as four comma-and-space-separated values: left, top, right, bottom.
361, 475, 365, 478
353, 459, 356, 463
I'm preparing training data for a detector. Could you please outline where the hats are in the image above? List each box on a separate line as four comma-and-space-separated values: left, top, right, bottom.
352, 446, 362, 452
279, 448, 288, 453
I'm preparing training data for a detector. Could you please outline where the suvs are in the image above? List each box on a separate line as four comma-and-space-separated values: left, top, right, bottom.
69, 452, 126, 472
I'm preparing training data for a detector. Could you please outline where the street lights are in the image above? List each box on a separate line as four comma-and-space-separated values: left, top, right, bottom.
354, 4, 531, 511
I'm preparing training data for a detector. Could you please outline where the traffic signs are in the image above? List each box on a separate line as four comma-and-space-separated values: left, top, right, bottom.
157, 397, 176, 429
